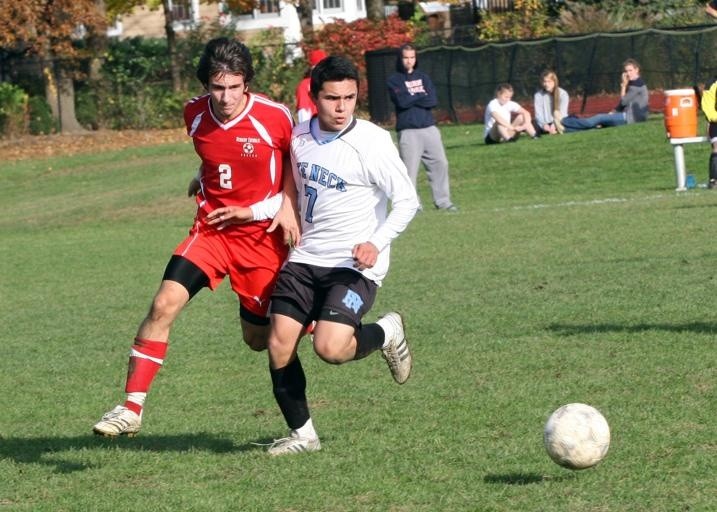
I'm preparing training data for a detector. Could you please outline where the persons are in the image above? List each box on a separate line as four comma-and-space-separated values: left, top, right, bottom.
385, 42, 457, 213
552, 58, 650, 136
89, 38, 319, 438
701, 79, 716, 189
293, 48, 332, 121
534, 69, 581, 136
482, 83, 541, 144
263, 55, 419, 456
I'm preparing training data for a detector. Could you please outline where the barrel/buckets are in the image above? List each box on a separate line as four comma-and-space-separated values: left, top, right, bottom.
663, 88, 698, 138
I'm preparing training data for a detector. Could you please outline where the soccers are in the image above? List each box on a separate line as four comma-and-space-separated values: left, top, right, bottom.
544, 403, 611, 470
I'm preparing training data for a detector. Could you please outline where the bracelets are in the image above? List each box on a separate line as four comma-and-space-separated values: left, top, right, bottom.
195, 169, 202, 182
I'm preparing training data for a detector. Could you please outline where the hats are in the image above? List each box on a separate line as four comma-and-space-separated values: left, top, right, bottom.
309, 49, 327, 68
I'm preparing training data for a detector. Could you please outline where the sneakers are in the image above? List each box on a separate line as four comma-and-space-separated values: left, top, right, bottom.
375, 311, 411, 384
249, 432, 321, 456
708, 178, 716, 189
92, 404, 142, 436
439, 203, 456, 212
